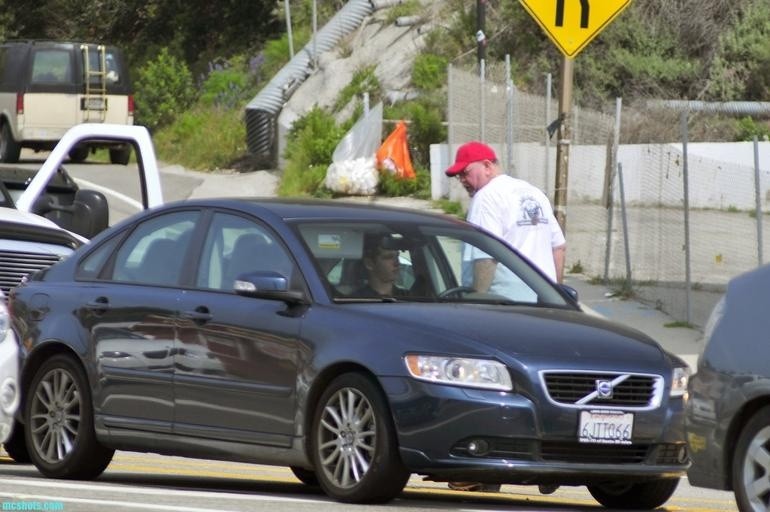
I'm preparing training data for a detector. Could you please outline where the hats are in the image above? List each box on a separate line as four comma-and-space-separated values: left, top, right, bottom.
446, 142, 496, 176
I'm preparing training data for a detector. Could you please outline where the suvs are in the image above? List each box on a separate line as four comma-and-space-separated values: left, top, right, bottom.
0, 37, 138, 165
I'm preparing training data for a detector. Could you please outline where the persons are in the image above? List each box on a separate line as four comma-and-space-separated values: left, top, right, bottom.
346, 231, 414, 302
444, 141, 567, 496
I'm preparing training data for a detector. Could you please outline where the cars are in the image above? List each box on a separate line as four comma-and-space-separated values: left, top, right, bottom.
0, 122, 770, 512
